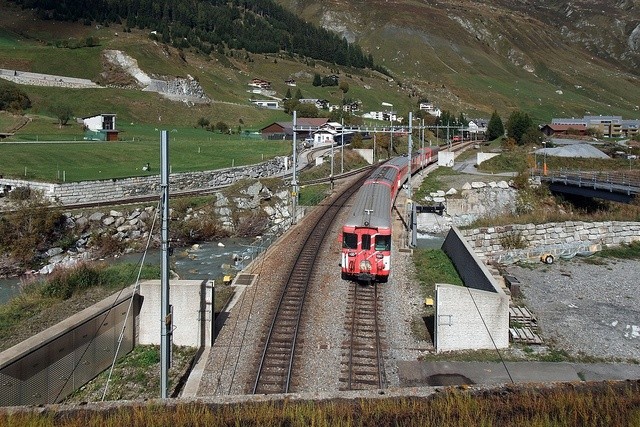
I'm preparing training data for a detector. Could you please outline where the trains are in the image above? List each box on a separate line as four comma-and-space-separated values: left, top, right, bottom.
339, 145, 440, 282
453, 134, 464, 141
